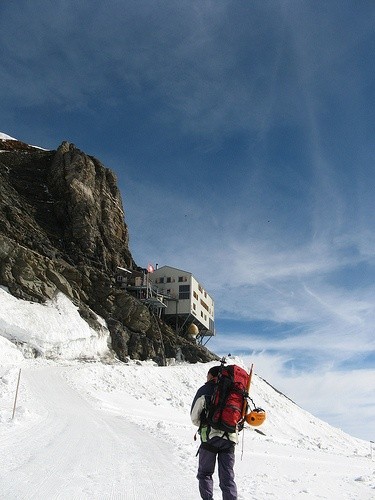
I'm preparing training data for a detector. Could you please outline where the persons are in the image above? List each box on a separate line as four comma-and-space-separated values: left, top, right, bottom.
190, 366, 239, 500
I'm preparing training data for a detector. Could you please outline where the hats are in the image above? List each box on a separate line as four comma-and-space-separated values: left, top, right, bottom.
208, 366, 221, 376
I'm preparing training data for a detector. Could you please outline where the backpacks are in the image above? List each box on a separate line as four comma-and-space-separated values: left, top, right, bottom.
188, 324, 198, 333
207, 365, 250, 433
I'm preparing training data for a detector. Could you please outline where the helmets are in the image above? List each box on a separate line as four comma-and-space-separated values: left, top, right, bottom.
246, 408, 266, 426
